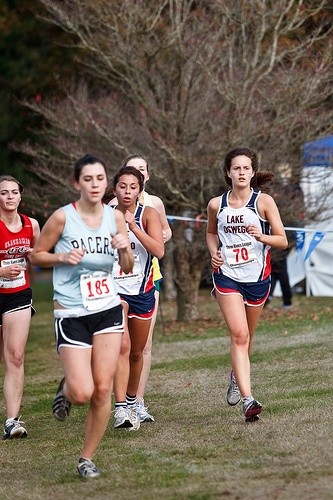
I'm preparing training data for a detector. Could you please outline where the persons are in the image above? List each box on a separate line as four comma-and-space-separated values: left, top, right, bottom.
31, 155, 135, 479
109, 166, 165, 433
261, 222, 295, 311
206, 148, 289, 422
108, 156, 172, 423
0, 176, 41, 438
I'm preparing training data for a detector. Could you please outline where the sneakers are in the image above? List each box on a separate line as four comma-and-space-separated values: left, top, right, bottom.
4, 414, 27, 439
112, 406, 133, 428
78, 457, 100, 479
124, 405, 140, 432
241, 396, 262, 423
52, 376, 72, 422
226, 370, 241, 405
136, 400, 154, 422
